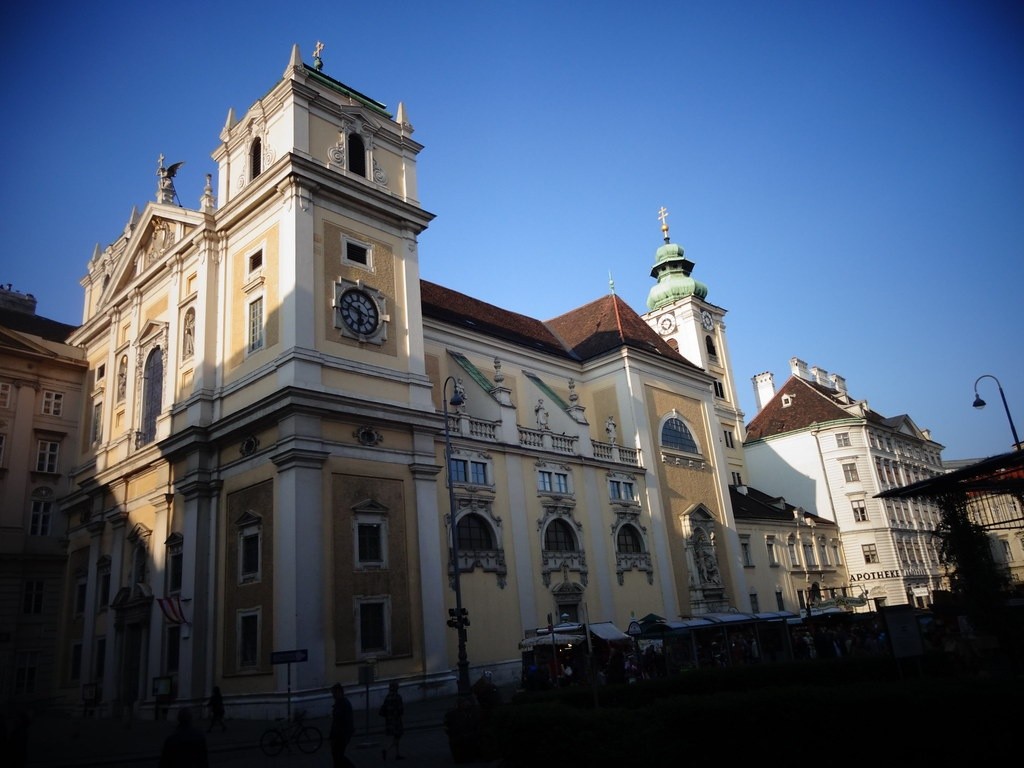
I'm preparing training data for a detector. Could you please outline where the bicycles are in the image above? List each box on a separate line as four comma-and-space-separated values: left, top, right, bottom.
260, 707, 324, 755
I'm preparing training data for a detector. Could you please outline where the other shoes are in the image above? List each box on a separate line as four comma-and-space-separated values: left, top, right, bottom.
395, 756, 406, 761
380, 749, 388, 762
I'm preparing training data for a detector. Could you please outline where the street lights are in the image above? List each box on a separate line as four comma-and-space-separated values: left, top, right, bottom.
971, 373, 1023, 453
443, 374, 472, 685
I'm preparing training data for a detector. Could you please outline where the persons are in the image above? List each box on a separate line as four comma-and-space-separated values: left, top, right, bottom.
520, 611, 974, 694
329, 683, 356, 768
205, 685, 227, 733
380, 682, 405, 763
156, 706, 209, 768
